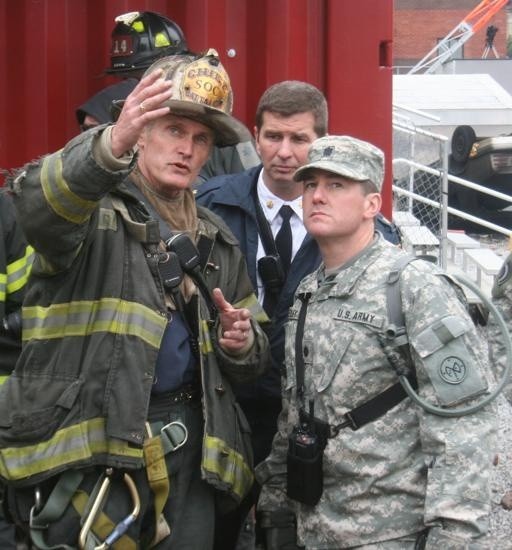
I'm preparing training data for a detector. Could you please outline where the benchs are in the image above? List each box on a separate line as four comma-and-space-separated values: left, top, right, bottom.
393, 210, 503, 289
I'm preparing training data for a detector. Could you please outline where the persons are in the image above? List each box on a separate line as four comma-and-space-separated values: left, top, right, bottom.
101, 9, 263, 186
254, 131, 501, 550
193, 79, 407, 550
0, 52, 276, 550
0, 190, 37, 387
74, 77, 139, 134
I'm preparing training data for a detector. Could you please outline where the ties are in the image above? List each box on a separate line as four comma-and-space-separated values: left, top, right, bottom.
261, 206, 293, 319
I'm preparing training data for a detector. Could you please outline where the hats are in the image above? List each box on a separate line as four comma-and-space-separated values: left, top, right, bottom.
294, 134, 386, 192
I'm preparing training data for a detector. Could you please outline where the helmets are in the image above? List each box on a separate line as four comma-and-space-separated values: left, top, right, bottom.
111, 48, 252, 148
104, 11, 186, 76
